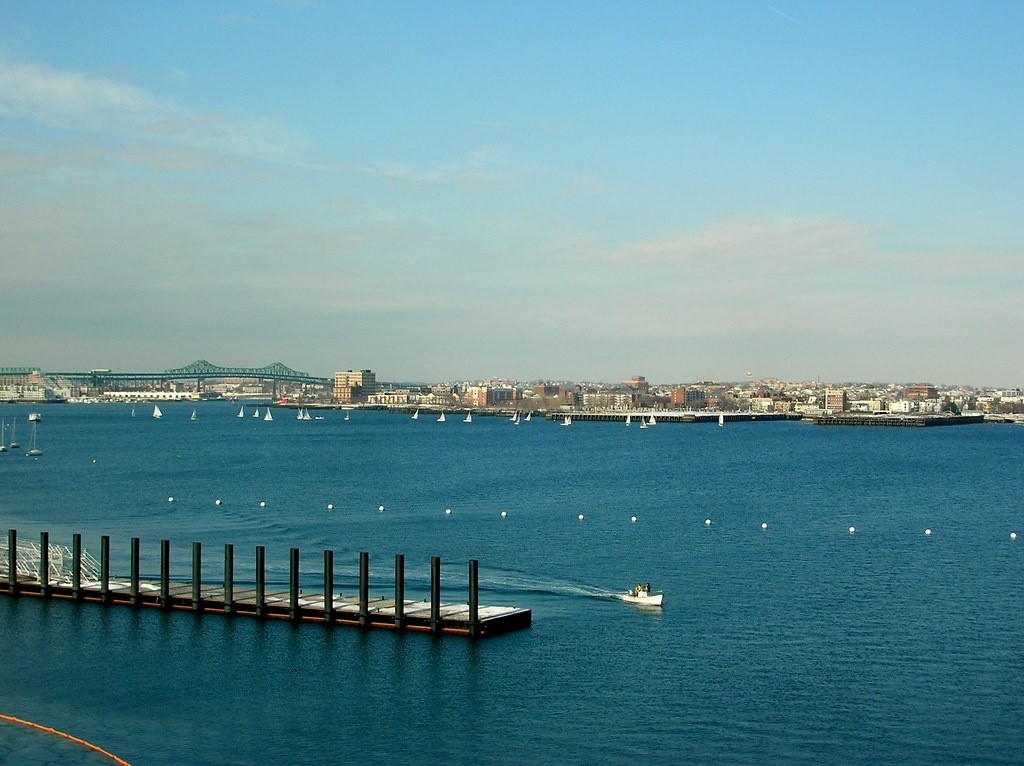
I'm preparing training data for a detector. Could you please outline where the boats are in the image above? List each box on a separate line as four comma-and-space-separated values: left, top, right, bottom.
622, 591, 664, 606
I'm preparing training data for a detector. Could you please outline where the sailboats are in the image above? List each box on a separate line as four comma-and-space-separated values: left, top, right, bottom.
0, 409, 725, 460
